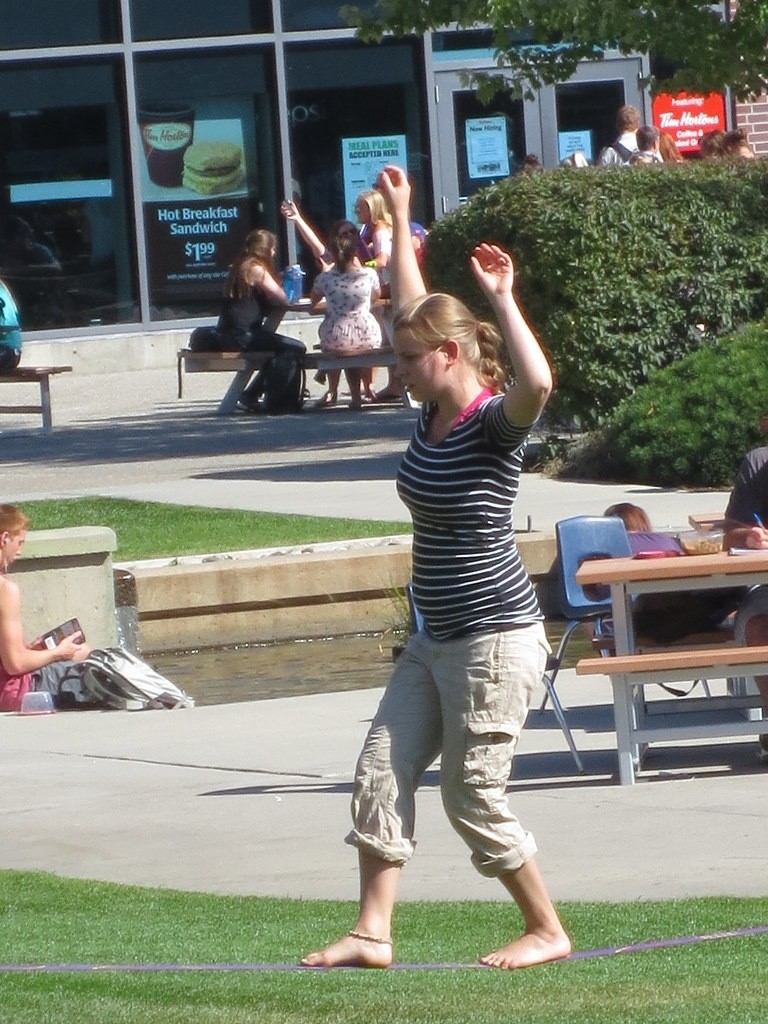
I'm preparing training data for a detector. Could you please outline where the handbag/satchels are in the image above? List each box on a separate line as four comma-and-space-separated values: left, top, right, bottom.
188, 326, 221, 353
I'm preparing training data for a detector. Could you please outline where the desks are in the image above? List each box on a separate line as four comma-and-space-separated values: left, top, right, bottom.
574, 548, 767, 766
289, 296, 391, 315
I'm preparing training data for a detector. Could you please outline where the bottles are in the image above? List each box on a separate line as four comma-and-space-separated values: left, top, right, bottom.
284, 264, 303, 302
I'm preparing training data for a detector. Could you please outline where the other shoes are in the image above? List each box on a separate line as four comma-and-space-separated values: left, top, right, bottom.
236, 391, 264, 413
373, 388, 403, 403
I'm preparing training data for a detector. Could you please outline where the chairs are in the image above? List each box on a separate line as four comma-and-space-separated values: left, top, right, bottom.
535, 515, 711, 716
404, 582, 585, 775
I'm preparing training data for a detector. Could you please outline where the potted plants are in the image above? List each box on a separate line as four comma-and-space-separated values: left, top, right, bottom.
372, 571, 413, 665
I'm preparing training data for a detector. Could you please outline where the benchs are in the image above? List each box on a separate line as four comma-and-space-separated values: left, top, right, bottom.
0, 366, 72, 428
575, 646, 767, 786
178, 344, 411, 409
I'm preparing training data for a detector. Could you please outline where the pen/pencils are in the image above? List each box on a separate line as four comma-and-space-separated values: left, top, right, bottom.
751, 512, 768, 529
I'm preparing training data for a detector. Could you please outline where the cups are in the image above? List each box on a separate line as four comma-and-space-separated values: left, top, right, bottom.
138, 103, 194, 185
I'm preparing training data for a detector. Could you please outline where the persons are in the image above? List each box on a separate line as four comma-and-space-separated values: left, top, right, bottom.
582, 503, 681, 650
217, 191, 429, 412
301, 165, 574, 970
722, 413, 768, 766
0, 503, 91, 712
517, 105, 756, 172
0, 279, 22, 369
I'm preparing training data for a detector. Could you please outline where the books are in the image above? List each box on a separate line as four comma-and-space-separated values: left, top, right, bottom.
728, 547, 768, 555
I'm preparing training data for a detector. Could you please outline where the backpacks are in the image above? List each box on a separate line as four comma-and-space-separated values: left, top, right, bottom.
59, 646, 194, 711
264, 363, 311, 415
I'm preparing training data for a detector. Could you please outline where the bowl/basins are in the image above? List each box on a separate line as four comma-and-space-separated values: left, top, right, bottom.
21, 692, 53, 715
680, 528, 724, 555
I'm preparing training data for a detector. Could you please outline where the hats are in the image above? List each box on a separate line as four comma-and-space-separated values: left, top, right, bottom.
410, 222, 427, 240
